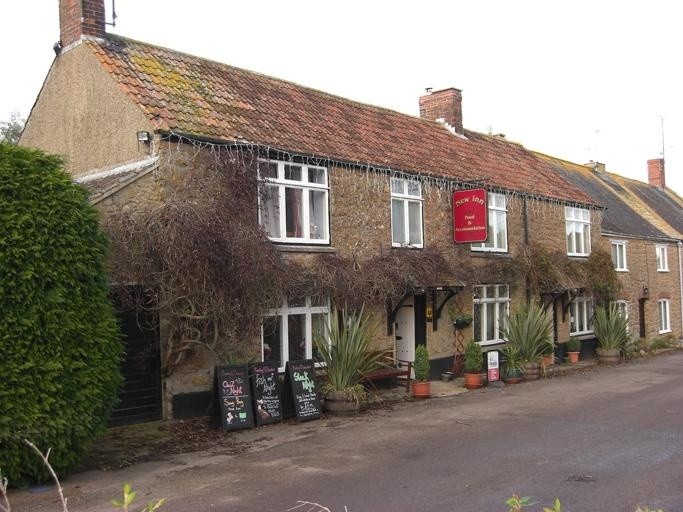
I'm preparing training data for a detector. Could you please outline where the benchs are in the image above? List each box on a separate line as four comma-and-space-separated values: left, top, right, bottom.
357, 357, 412, 393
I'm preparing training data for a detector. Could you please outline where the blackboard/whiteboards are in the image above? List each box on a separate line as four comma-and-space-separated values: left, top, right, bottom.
285, 360, 322, 422
252, 363, 283, 425
212, 364, 255, 429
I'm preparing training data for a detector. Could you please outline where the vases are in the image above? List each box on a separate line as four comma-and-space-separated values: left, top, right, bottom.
440, 371, 454, 382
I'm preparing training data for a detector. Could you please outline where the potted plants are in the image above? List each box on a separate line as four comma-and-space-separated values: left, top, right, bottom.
502, 345, 521, 385
589, 301, 633, 364
461, 340, 484, 391
410, 342, 432, 400
311, 300, 399, 416
565, 337, 583, 364
499, 297, 559, 380
539, 341, 554, 366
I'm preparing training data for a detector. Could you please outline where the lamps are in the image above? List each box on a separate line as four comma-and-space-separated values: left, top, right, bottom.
135, 130, 150, 144
